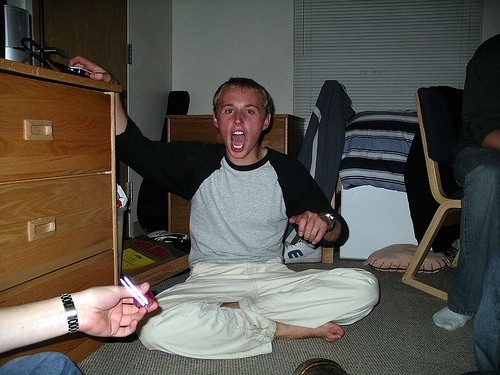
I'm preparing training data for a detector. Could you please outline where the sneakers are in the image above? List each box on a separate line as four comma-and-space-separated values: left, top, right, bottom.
283, 240, 322, 263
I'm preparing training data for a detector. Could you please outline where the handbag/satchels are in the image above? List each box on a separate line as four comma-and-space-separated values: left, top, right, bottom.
121, 234, 191, 287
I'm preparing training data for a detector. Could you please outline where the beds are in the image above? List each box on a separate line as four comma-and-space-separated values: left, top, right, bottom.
299, 80, 418, 264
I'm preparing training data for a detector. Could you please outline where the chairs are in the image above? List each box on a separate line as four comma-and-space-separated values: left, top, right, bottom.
401, 84, 463, 301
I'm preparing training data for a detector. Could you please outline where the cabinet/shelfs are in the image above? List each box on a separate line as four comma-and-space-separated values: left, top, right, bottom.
0, 59, 124, 366
165, 114, 305, 237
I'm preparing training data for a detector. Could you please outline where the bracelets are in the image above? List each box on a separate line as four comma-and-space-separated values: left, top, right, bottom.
69, 55, 379, 359
317, 212, 336, 233
60, 292, 79, 333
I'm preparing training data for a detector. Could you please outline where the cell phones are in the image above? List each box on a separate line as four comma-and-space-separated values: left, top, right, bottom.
67, 66, 92, 77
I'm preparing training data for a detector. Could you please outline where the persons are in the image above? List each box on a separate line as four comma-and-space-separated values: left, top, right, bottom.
292, 33, 500, 375
0, 282, 158, 375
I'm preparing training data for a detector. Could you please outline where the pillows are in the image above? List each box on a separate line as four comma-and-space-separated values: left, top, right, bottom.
367, 243, 451, 274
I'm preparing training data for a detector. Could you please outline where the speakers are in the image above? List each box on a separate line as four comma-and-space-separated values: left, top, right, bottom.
0, 4, 32, 64
